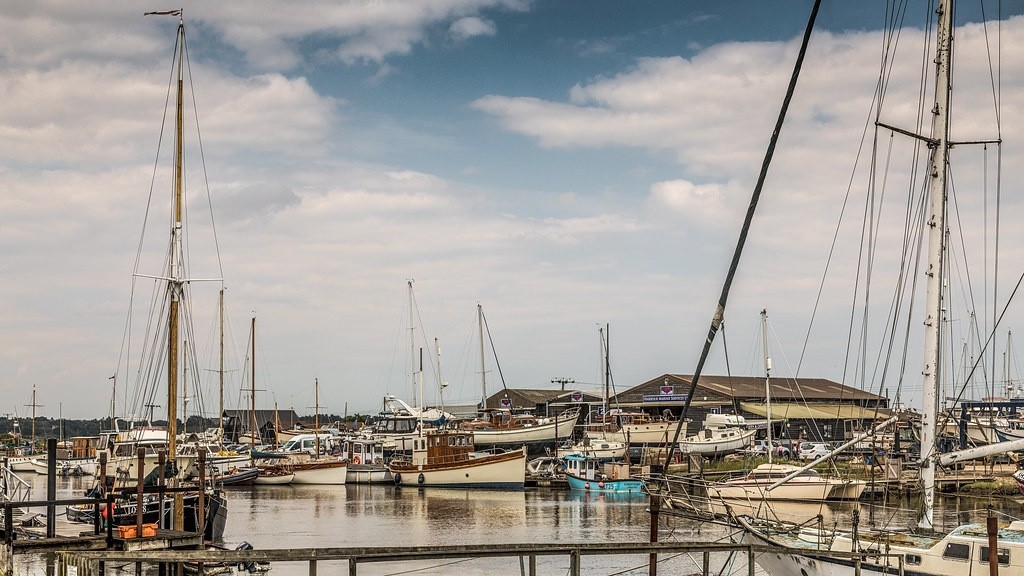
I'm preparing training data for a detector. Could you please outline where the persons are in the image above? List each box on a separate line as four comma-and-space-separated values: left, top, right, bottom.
795, 440, 802, 462
229, 466, 239, 475
772, 441, 784, 459
537, 416, 551, 426
663, 408, 674, 422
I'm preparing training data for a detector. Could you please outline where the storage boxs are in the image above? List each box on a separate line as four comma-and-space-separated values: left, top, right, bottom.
119, 523, 158, 538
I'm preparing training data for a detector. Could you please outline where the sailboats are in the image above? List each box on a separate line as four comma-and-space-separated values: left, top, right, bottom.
0, 0, 1024, 575
